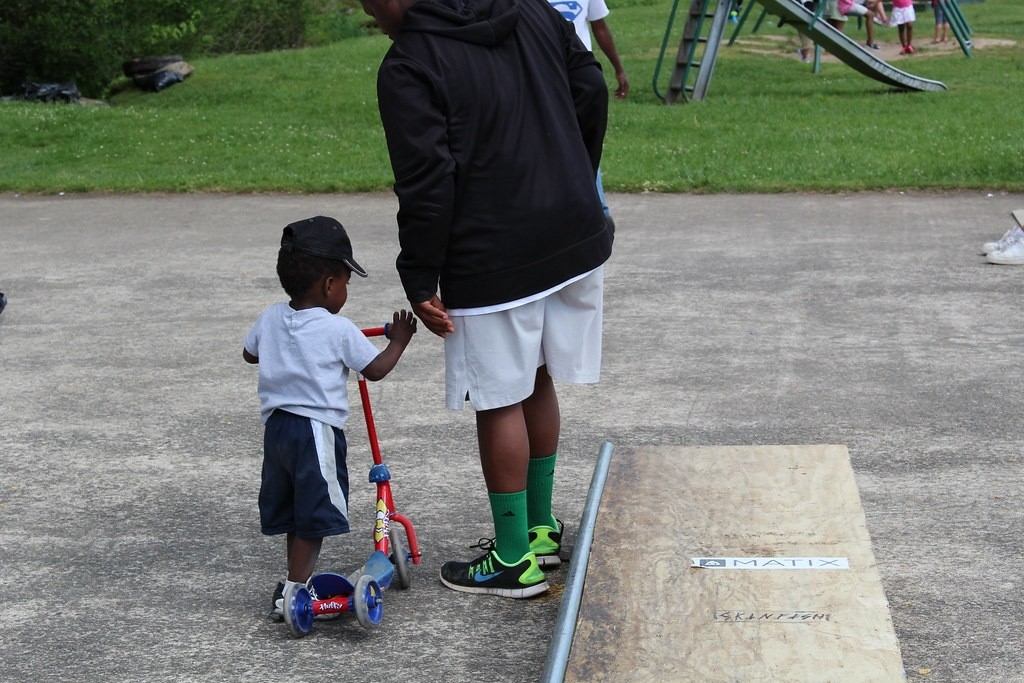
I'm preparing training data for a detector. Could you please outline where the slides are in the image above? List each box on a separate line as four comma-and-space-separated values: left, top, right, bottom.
758, 0, 948, 92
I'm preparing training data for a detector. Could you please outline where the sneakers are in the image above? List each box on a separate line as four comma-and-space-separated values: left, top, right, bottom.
980, 225, 1024, 265
526, 512, 565, 565
439, 537, 550, 598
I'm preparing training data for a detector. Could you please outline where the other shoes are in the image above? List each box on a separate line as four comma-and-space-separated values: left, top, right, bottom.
797, 47, 810, 64
907, 45, 914, 54
899, 47, 907, 54
872, 17, 897, 28
865, 39, 880, 49
267, 581, 340, 621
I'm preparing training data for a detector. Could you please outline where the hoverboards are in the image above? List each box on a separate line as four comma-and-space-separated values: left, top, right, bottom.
283, 324, 422, 640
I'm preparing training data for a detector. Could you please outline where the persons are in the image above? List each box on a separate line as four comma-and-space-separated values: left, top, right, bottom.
360, 0, 628, 600
728, 0, 743, 20
242, 215, 417, 623
980, 210, 1024, 266
932, 0, 949, 43
797, 0, 917, 64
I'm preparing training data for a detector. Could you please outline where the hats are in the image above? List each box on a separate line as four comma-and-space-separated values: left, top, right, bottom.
281, 216, 369, 278
